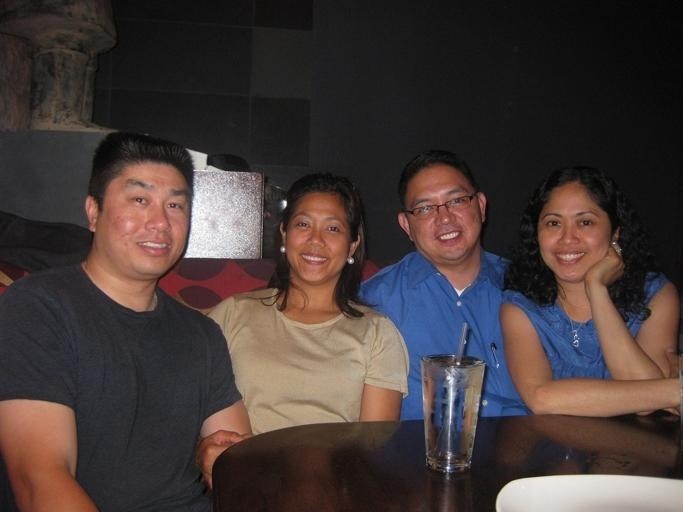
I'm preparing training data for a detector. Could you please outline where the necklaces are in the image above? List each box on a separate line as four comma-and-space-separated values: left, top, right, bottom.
564, 287, 595, 351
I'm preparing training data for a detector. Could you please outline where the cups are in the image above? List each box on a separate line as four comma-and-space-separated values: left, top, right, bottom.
422, 353, 487, 477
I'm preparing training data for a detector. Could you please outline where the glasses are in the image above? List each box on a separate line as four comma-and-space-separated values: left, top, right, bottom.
401, 192, 477, 218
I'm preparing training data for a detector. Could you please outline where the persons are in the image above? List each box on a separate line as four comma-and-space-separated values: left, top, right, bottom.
500, 165, 682, 425
360, 153, 547, 417
0, 129, 259, 510
199, 171, 406, 455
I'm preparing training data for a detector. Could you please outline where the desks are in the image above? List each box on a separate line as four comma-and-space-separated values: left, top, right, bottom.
212, 410, 683, 512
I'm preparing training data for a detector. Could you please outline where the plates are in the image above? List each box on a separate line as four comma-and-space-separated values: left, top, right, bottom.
492, 474, 681, 512
